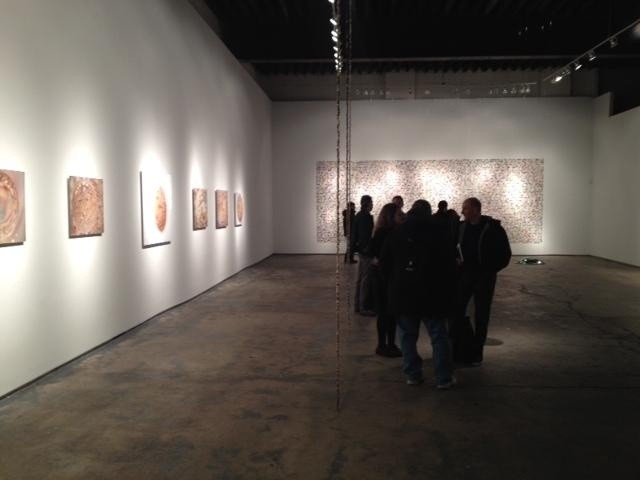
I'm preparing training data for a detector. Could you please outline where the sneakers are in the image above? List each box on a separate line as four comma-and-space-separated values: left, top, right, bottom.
434, 376, 456, 389
406, 375, 425, 385
376, 344, 402, 358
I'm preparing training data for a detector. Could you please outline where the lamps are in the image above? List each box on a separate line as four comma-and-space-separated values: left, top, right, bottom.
350, 81, 539, 96
328, 0, 344, 75
548, 36, 618, 85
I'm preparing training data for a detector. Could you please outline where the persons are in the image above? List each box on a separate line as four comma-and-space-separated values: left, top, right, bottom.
341, 193, 511, 391
